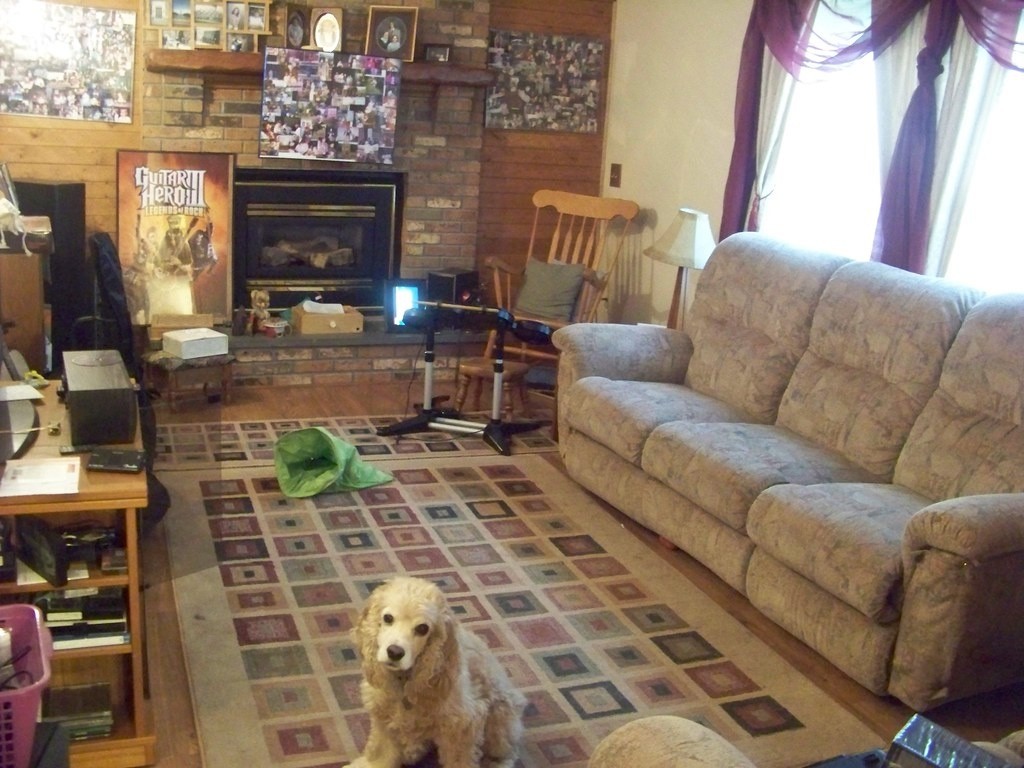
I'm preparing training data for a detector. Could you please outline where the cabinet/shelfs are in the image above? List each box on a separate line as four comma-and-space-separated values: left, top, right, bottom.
0, 379, 157, 768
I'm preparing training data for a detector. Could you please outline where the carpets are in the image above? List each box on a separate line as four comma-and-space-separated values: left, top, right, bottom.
152, 408, 560, 472
155, 454, 887, 768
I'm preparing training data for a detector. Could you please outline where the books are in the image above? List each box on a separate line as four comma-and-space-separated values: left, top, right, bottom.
42, 597, 131, 651
35, 682, 114, 740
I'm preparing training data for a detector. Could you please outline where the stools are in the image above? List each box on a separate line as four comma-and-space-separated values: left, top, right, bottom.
140, 351, 237, 412
454, 358, 535, 420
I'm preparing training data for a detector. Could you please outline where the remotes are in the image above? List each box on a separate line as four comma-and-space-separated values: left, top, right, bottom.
59, 445, 94, 453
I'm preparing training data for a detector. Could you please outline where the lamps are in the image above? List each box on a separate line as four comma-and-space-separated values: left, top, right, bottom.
641, 208, 717, 330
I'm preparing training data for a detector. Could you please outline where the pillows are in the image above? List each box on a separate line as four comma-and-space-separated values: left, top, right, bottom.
551, 258, 610, 323
515, 257, 585, 322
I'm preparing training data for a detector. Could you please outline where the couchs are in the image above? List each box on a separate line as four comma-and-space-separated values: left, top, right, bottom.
550, 229, 1024, 713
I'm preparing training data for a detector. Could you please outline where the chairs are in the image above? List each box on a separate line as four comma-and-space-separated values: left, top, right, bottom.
472, 189, 639, 412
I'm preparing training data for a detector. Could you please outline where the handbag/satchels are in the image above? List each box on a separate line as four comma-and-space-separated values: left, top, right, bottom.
272, 425, 394, 498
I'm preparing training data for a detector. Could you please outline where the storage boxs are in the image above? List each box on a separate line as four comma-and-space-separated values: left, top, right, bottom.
148, 312, 214, 342
162, 327, 229, 359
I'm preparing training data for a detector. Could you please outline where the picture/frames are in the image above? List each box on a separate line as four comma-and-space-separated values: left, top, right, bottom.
364, 4, 419, 62
142, 0, 273, 53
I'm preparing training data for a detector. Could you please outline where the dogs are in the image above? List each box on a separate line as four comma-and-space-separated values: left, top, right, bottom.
342, 574, 529, 768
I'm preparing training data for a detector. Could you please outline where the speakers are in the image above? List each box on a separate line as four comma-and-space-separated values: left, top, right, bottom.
428, 267, 479, 327
61, 350, 138, 445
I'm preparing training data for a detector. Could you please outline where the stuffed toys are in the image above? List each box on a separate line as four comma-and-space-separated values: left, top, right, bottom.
245, 289, 271, 336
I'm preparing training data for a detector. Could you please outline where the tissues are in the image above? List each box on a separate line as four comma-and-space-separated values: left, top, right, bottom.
291, 299, 364, 334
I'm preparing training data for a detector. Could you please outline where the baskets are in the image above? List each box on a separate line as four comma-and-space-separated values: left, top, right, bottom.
0, 603, 52, 768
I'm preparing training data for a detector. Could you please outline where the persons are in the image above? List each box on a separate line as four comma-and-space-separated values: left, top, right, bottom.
288, 17, 303, 44
264, 48, 397, 164
228, 6, 240, 29
380, 22, 401, 51
249, 12, 263, 27
0, 0, 131, 123
486, 31, 600, 133
